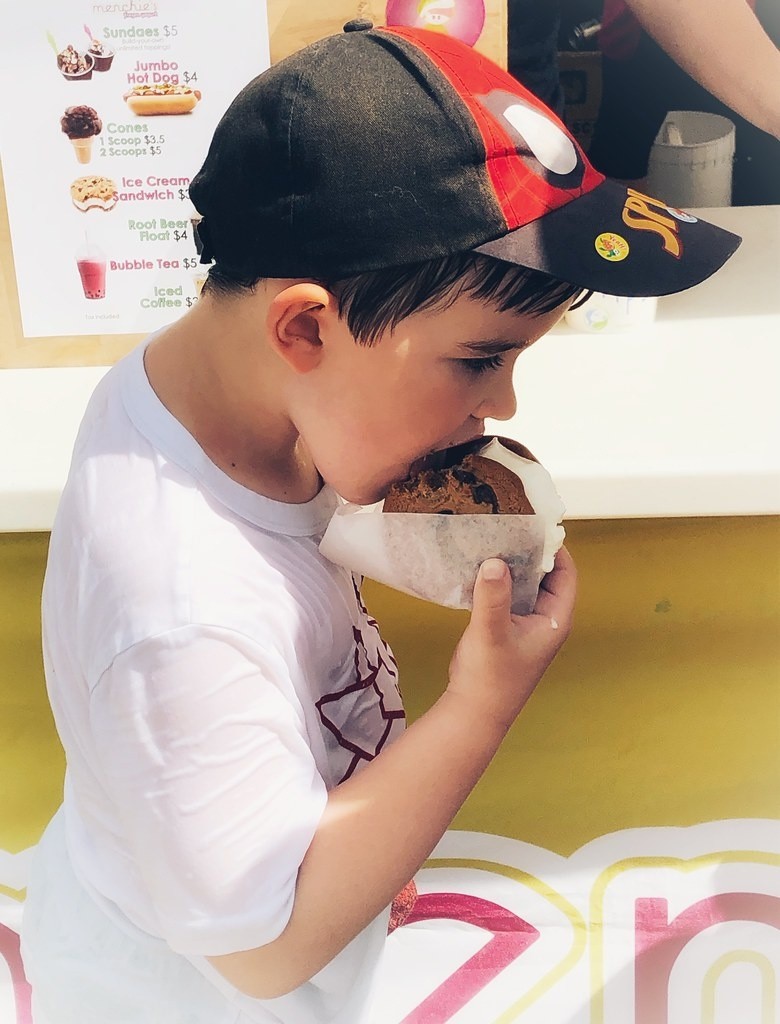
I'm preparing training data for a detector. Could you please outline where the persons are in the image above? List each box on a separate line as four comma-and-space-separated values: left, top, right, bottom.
585, 0, 780, 208
508, 0, 565, 117
22, 20, 744, 1023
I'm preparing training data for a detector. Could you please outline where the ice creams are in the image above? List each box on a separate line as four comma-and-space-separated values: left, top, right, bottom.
61, 106, 103, 165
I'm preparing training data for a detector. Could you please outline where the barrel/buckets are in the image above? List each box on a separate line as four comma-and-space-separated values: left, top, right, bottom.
646, 112, 736, 207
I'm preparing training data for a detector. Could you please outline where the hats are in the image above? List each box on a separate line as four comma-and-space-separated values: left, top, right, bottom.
189, 19, 742, 297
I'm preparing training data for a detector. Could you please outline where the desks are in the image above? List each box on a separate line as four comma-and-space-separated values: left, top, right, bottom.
0, 201, 780, 1024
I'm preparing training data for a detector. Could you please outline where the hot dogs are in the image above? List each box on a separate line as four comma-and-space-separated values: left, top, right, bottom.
122, 85, 202, 115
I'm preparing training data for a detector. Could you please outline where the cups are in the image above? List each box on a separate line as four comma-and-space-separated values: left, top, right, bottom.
56, 49, 94, 80
86, 45, 114, 71
73, 253, 107, 298
72, 135, 92, 163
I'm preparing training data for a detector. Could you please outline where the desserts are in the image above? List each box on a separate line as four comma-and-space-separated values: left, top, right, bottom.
72, 176, 118, 210
382, 435, 566, 574
